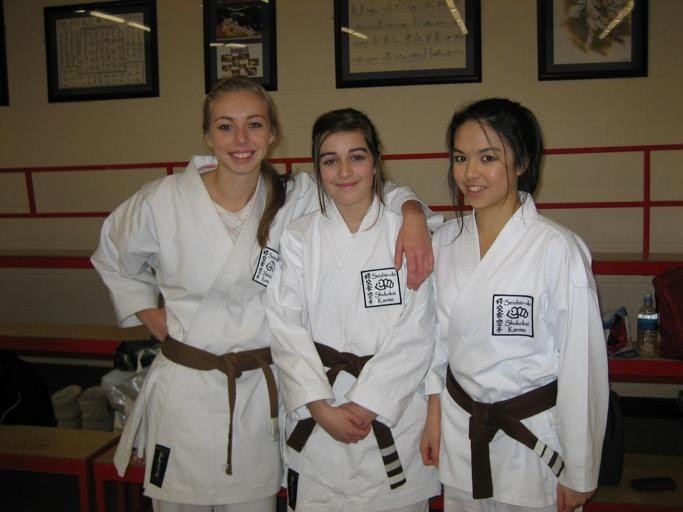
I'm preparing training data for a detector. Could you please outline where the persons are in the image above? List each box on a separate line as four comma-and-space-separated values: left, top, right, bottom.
260, 106, 441, 512
419, 97, 611, 512
88, 74, 445, 512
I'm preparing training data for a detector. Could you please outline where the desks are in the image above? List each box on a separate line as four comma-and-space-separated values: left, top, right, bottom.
607, 344, 683, 381
0, 319, 152, 358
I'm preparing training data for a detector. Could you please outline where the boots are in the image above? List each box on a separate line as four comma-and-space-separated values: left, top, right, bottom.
50, 383, 109, 432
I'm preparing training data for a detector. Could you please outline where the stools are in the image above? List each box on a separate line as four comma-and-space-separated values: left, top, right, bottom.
2, 424, 147, 510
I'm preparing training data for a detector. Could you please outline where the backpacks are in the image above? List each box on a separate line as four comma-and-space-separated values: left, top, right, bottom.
653, 266, 683, 362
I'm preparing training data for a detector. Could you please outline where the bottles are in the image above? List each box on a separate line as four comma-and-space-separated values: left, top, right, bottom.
635, 294, 660, 358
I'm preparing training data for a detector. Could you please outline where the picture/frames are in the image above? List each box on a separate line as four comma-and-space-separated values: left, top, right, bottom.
535, 0, 648, 80
332, 0, 484, 90
44, 1, 159, 99
204, 0, 279, 92
